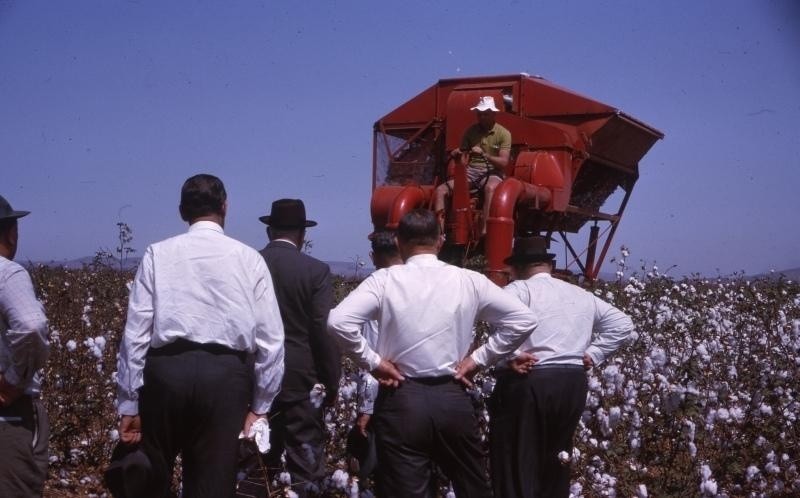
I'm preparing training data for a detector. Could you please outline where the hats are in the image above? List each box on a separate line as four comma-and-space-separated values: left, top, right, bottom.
256, 199, 317, 226
504, 236, 557, 263
471, 97, 499, 111
0, 195, 29, 220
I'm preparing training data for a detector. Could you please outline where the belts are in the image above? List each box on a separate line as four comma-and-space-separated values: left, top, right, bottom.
526, 364, 585, 369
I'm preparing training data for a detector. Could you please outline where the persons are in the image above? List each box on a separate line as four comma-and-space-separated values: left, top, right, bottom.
327, 208, 540, 497
116, 172, 286, 496
355, 226, 440, 495
486, 233, 634, 497
432, 95, 512, 238
253, 198, 343, 496
1, 195, 52, 496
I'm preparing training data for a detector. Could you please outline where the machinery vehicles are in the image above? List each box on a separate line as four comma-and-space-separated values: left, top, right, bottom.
366, 74, 666, 293
